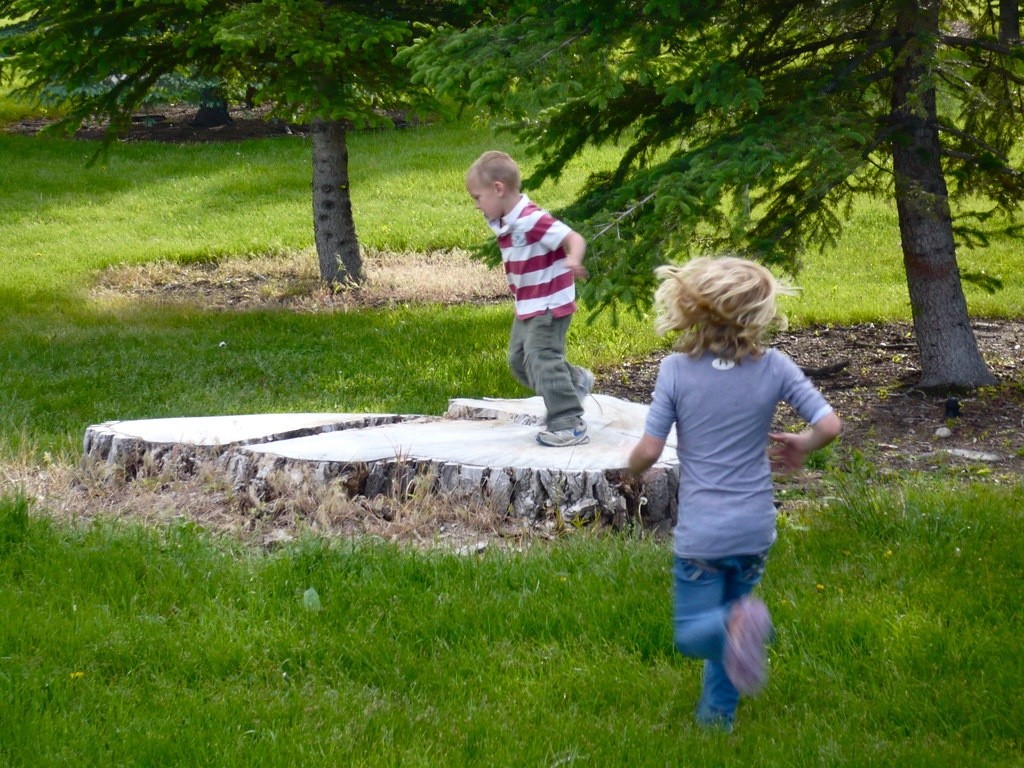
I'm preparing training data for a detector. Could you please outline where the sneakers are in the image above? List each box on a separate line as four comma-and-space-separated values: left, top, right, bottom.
536, 418, 589, 447
574, 367, 603, 412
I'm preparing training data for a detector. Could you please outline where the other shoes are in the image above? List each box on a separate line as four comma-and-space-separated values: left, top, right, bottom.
726, 598, 771, 693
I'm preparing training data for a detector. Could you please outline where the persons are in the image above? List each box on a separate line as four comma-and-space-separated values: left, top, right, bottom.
627, 256, 841, 729
462, 150, 598, 446
